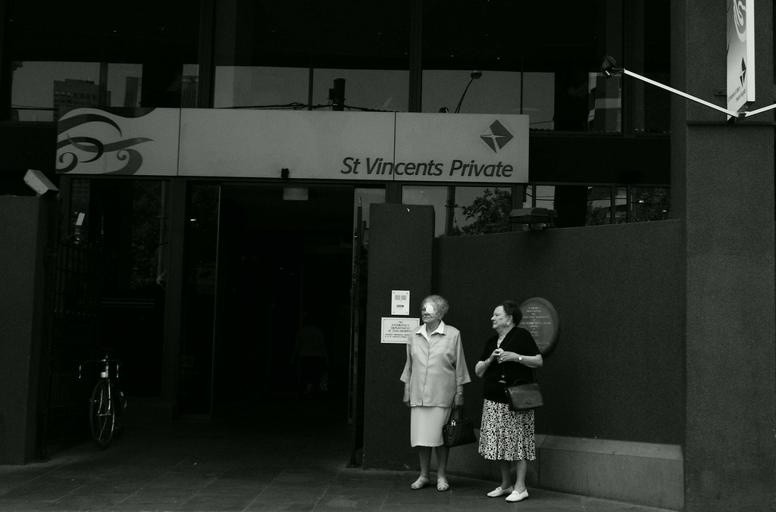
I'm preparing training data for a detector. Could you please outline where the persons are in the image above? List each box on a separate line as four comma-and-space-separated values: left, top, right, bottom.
473, 299, 543, 502
399, 295, 472, 492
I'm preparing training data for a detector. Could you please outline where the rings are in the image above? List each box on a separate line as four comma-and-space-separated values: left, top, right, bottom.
500, 357, 503, 361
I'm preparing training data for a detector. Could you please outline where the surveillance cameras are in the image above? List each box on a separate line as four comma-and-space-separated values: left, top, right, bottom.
22, 167, 58, 201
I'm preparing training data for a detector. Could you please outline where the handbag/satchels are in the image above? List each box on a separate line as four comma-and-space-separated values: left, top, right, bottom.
505, 383, 545, 412
443, 420, 478, 448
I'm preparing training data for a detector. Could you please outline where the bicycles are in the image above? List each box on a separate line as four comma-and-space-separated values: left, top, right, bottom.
77, 351, 131, 449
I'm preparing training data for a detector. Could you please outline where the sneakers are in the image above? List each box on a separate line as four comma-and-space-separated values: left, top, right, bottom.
437, 478, 450, 491
410, 476, 429, 490
486, 485, 529, 502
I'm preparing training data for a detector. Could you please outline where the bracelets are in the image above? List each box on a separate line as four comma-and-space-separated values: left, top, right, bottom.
518, 355, 522, 364
455, 390, 464, 396
